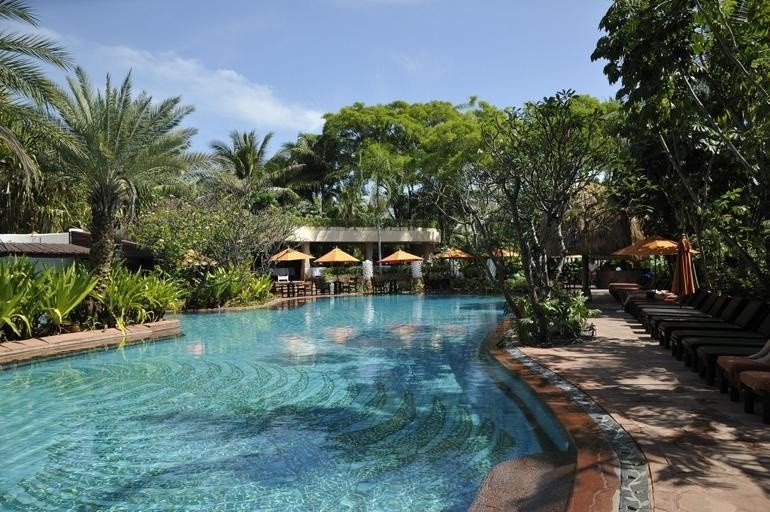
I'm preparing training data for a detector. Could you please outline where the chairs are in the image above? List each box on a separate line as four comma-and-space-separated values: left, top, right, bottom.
274, 274, 451, 298
608, 274, 770, 424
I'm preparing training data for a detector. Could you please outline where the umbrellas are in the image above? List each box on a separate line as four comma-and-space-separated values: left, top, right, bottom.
479, 248, 522, 260
266, 247, 317, 280
430, 246, 476, 261
376, 249, 426, 266
668, 232, 700, 307
312, 245, 361, 283
165, 249, 221, 269
607, 233, 704, 293
